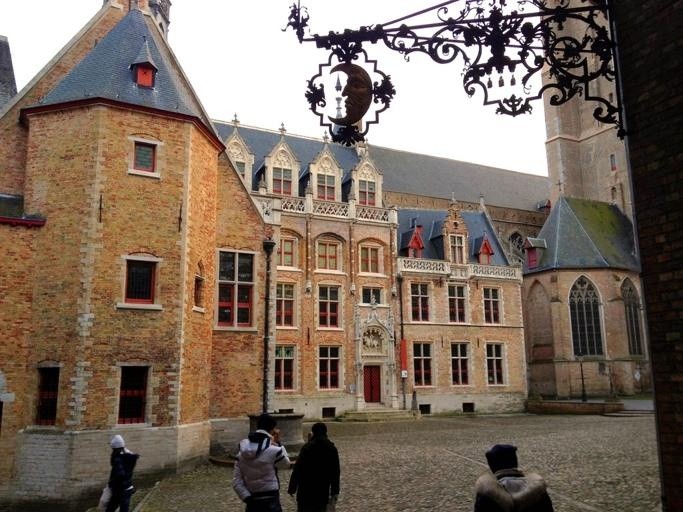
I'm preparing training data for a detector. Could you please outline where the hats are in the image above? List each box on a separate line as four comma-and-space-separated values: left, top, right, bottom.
109, 435, 125, 449
485, 445, 520, 473
255, 413, 277, 432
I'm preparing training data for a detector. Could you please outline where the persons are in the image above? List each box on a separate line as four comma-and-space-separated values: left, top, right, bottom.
470, 443, 556, 511
286, 422, 343, 511
231, 412, 289, 511
104, 434, 136, 512
341, 72, 373, 123
